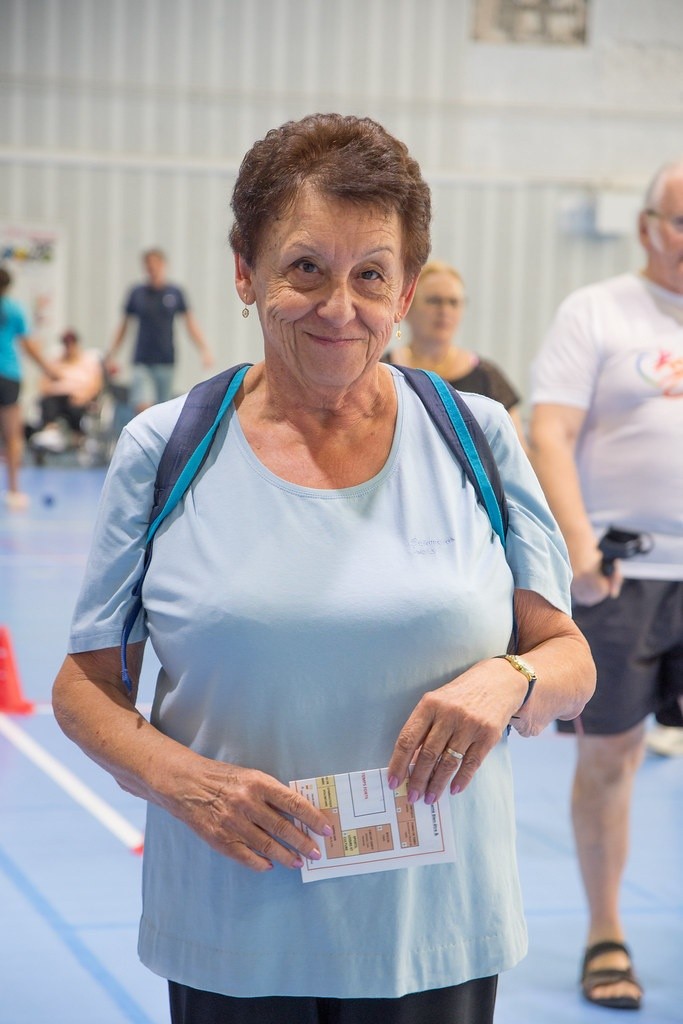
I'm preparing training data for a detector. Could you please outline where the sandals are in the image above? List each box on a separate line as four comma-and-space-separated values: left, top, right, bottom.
582, 940, 643, 1010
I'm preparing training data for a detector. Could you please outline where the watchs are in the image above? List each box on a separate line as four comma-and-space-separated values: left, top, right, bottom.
493, 653, 537, 719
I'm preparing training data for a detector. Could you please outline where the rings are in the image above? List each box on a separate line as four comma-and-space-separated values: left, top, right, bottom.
445, 747, 465, 762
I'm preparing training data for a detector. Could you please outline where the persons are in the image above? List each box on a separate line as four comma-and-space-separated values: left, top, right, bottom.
527, 158, 683, 1009
29, 248, 214, 454
0, 268, 63, 507
383, 257, 527, 452
51, 111, 597, 1023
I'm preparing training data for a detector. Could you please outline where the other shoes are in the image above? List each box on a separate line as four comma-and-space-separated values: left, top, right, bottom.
7, 492, 29, 511
643, 720, 683, 758
29, 429, 63, 454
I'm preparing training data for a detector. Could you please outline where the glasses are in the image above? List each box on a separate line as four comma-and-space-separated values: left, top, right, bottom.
647, 209, 683, 232
